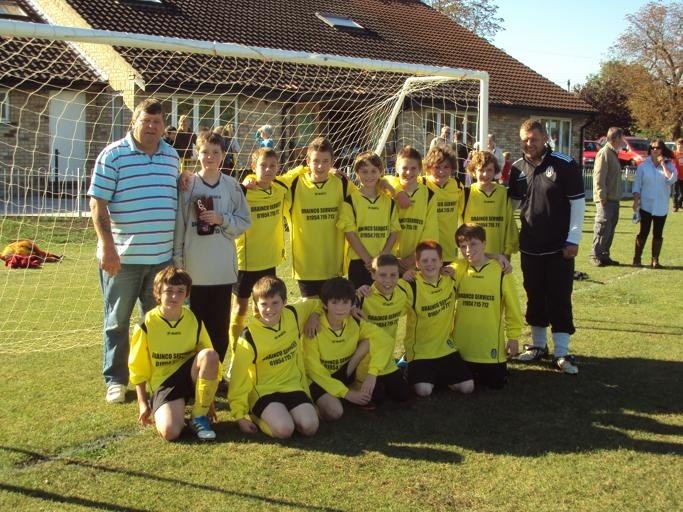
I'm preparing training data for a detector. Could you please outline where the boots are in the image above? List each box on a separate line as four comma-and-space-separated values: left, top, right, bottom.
632, 234, 647, 266
651, 236, 663, 269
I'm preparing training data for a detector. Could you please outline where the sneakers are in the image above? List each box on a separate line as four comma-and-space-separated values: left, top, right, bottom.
518, 344, 548, 362
106, 384, 127, 404
591, 257, 619, 266
186, 416, 216, 442
552, 355, 579, 374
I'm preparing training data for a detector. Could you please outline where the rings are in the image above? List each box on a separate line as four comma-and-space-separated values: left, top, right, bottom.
572, 254, 575, 258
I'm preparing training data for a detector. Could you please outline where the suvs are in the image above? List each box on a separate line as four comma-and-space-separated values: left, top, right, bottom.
597, 136, 650, 170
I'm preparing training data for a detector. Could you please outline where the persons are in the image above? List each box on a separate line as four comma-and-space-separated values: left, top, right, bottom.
87, 99, 180, 403
507, 120, 585, 374
162, 115, 242, 178
172, 131, 520, 363
226, 223, 523, 439
632, 139, 678, 268
590, 127, 625, 266
671, 138, 683, 212
128, 266, 222, 440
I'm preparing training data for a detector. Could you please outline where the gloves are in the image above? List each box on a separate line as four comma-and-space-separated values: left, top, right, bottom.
195, 196, 214, 235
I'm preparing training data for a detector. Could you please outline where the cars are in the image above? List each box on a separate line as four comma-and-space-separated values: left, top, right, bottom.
582, 140, 604, 169
663, 142, 677, 151
